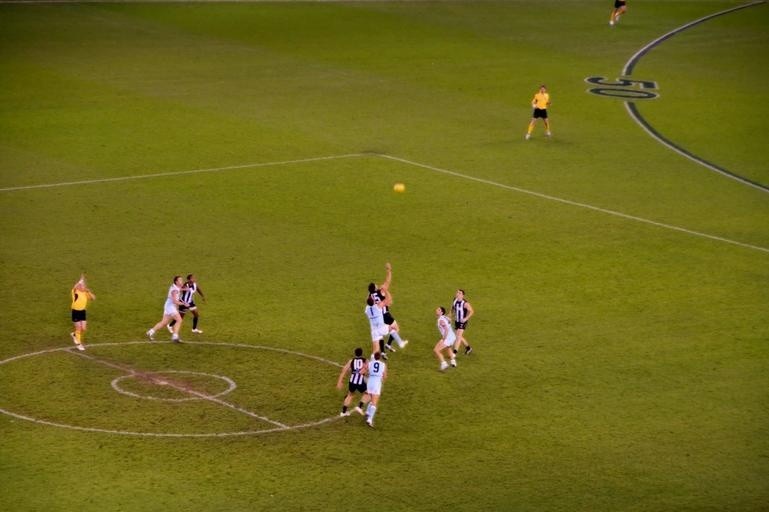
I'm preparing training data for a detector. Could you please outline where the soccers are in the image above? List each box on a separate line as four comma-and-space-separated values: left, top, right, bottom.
394, 183, 405, 192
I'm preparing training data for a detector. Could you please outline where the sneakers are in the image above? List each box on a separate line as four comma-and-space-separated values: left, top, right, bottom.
340, 407, 375, 429
371, 339, 408, 361
525, 131, 551, 140
69, 331, 85, 351
439, 347, 472, 371
146, 324, 203, 343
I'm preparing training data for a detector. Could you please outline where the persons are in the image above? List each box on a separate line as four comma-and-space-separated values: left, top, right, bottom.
145, 276, 192, 343
167, 273, 206, 334
70, 274, 96, 352
525, 85, 553, 139
336, 259, 473, 428
609, 0, 628, 26
